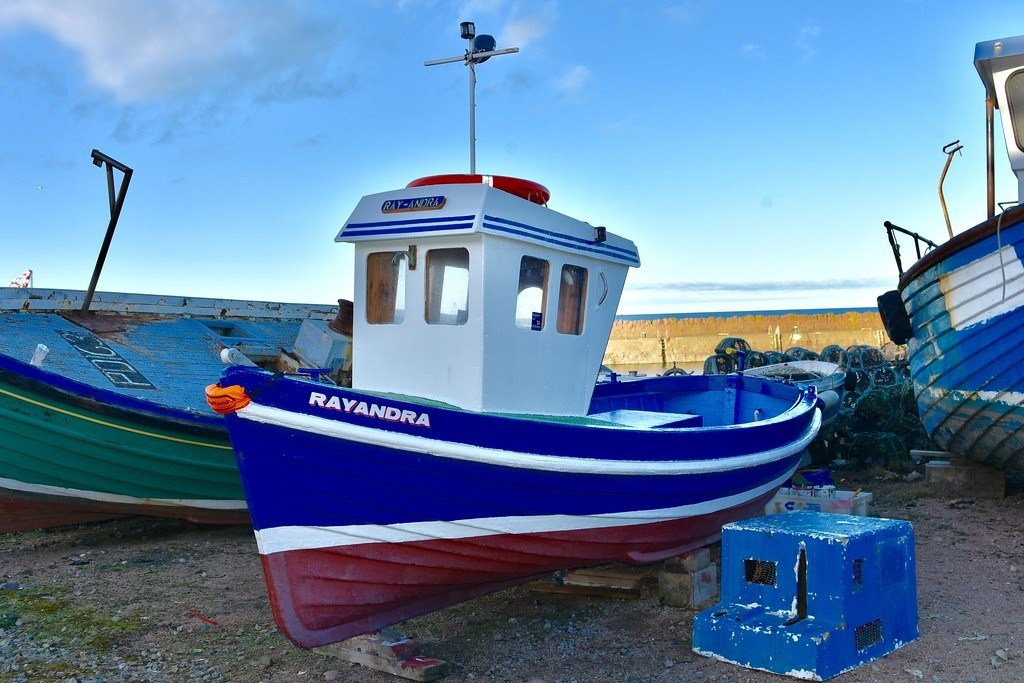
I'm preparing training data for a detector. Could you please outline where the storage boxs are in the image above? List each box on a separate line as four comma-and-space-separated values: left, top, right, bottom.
662, 549, 709, 572
765, 486, 873, 518
925, 462, 1005, 499
659, 562, 716, 607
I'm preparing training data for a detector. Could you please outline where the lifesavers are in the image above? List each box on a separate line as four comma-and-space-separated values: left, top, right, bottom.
876, 289, 914, 346
406, 173, 551, 207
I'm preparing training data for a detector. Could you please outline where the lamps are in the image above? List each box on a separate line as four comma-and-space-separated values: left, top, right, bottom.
563, 269, 585, 335
474, 35, 496, 63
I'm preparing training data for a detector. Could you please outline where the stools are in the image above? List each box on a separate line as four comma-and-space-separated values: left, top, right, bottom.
586, 409, 704, 427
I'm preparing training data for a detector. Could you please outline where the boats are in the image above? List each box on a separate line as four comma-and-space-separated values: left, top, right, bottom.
0, 149, 353, 533
205, 22, 846, 647
875, 34, 1024, 482
